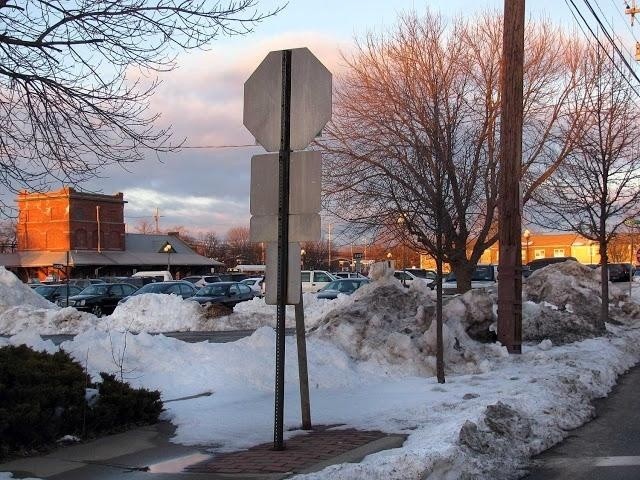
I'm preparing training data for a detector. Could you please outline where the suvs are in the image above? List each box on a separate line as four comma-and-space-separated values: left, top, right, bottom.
608, 263, 635, 283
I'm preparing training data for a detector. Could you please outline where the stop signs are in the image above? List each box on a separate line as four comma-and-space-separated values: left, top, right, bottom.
637, 247, 640, 263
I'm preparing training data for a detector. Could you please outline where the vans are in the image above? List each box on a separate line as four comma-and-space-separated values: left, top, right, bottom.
528, 256, 578, 271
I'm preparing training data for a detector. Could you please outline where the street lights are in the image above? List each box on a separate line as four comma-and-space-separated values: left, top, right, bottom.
524, 230, 531, 262
396, 211, 410, 282
386, 252, 392, 268
300, 249, 306, 271
587, 239, 595, 265
164, 244, 172, 271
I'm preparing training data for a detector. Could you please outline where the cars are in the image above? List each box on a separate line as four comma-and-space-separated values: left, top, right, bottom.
61, 283, 140, 318
31, 284, 85, 307
65, 270, 266, 282
182, 281, 261, 316
301, 264, 495, 300
118, 281, 199, 307
585, 264, 619, 280
